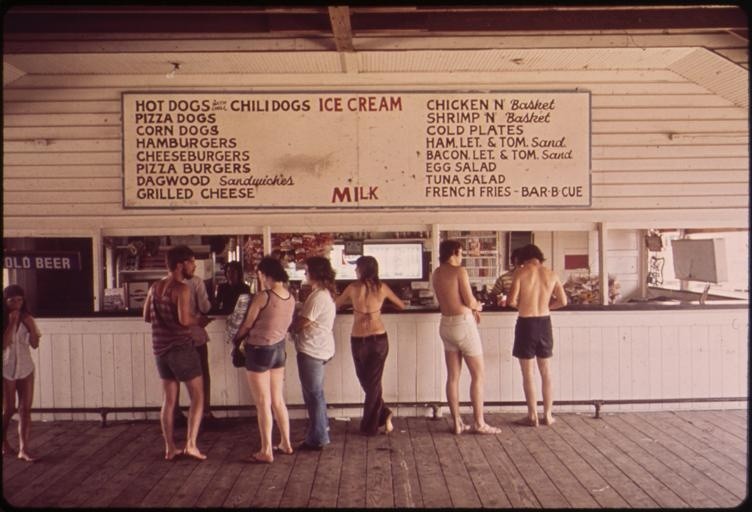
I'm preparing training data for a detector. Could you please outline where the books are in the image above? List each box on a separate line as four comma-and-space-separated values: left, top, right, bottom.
138, 254, 166, 268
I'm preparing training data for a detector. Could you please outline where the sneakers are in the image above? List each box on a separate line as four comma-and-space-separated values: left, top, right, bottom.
454, 423, 502, 436
299, 439, 325, 451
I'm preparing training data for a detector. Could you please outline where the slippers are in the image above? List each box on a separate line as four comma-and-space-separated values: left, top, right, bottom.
248, 444, 294, 464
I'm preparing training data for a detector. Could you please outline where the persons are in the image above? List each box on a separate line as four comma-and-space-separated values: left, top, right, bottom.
506, 244, 568, 427
230, 255, 298, 463
143, 245, 216, 460
3, 284, 42, 462
288, 255, 337, 449
489, 246, 557, 307
433, 239, 502, 435
215, 260, 251, 315
335, 256, 407, 436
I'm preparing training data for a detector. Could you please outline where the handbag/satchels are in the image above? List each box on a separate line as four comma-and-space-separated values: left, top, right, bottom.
232, 346, 246, 367
